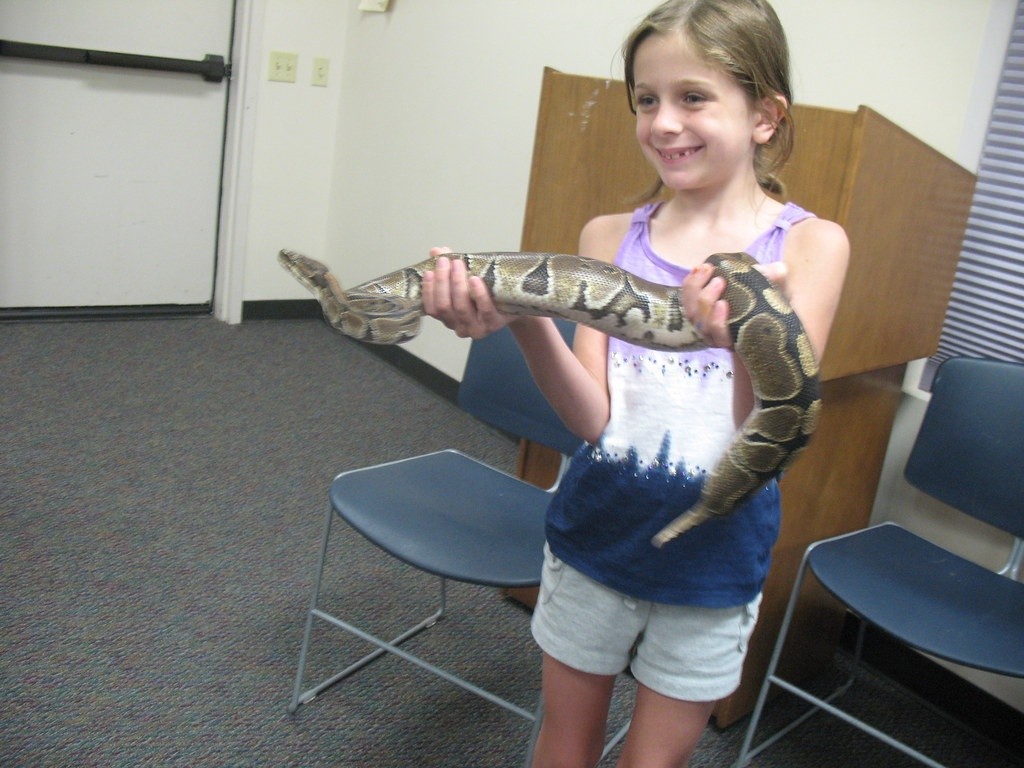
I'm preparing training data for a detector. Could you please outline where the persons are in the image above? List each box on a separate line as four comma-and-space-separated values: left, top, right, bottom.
421, 0, 850, 768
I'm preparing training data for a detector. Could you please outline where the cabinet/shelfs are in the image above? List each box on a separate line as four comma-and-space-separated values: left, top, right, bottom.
511, 64, 979, 729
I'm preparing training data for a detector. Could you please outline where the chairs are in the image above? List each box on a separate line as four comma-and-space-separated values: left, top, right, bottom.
726, 356, 1024, 768
286, 316, 634, 768
